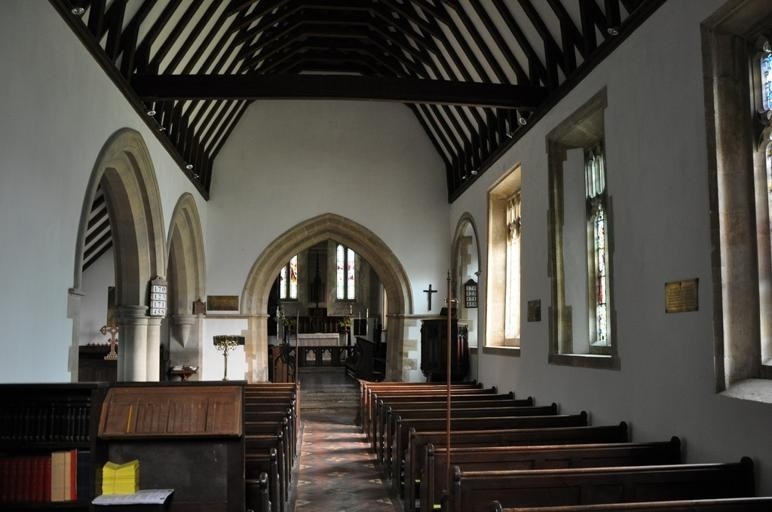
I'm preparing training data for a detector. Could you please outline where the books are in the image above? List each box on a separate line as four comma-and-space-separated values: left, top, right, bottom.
0, 395, 176, 511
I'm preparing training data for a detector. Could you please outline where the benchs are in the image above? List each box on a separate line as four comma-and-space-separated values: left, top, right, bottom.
353, 377, 770, 510
0, 376, 301, 511
